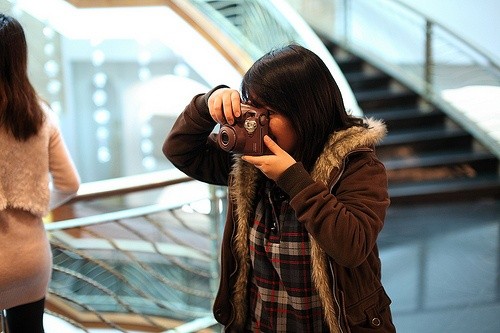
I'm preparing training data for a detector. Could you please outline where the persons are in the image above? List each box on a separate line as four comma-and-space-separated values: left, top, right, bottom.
162, 43, 395, 333
0, 11, 80, 333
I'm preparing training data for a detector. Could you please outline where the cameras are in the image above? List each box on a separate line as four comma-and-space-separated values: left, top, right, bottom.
218, 103, 270, 156
